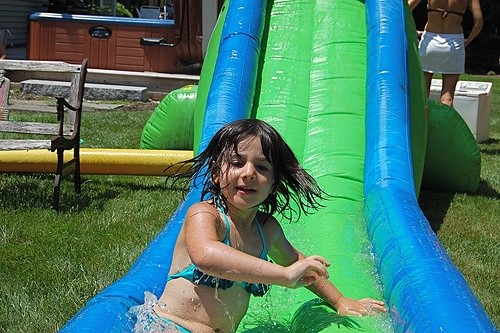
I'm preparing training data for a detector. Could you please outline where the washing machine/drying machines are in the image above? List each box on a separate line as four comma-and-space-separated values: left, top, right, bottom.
429, 79, 493, 143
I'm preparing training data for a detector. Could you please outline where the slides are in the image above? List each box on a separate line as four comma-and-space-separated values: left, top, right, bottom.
52, 2, 498, 332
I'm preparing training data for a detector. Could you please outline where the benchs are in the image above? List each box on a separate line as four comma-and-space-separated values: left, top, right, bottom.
0, 58, 89, 210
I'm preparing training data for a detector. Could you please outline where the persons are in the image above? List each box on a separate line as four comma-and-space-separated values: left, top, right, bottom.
139, 119, 387, 333
407, 0, 483, 108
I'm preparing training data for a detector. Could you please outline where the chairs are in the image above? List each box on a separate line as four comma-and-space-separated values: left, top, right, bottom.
140, 6, 160, 19
